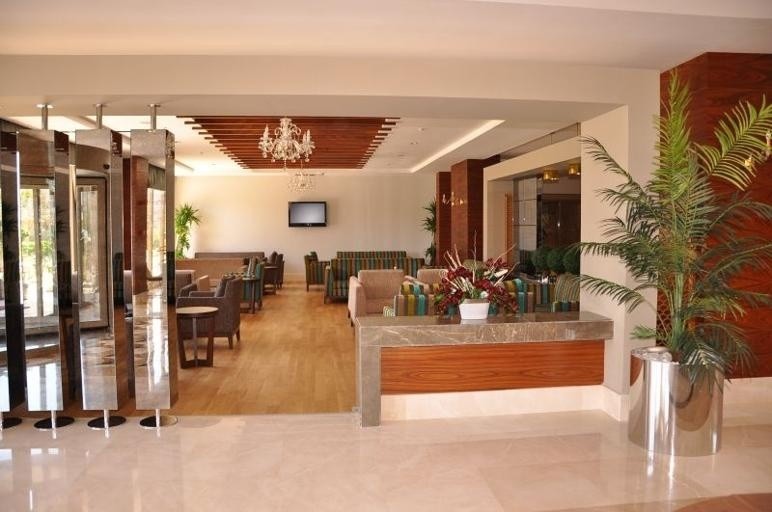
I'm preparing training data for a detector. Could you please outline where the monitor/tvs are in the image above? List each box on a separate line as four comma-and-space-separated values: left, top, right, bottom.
287, 201, 327, 228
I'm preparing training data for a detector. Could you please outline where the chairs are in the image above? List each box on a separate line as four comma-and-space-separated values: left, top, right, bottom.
176, 253, 285, 349
347, 268, 413, 325
415, 268, 451, 294
304, 252, 330, 291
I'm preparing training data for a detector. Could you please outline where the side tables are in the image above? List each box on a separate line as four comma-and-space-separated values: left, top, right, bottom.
175, 306, 218, 369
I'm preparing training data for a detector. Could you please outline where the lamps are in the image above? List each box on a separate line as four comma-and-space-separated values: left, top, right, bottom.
287, 160, 314, 193
258, 117, 316, 175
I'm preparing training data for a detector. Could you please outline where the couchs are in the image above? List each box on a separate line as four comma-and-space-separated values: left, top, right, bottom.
382, 278, 535, 317
325, 251, 421, 298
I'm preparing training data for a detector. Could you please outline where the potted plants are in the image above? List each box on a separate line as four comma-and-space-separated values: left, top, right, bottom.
553, 64, 772, 455
